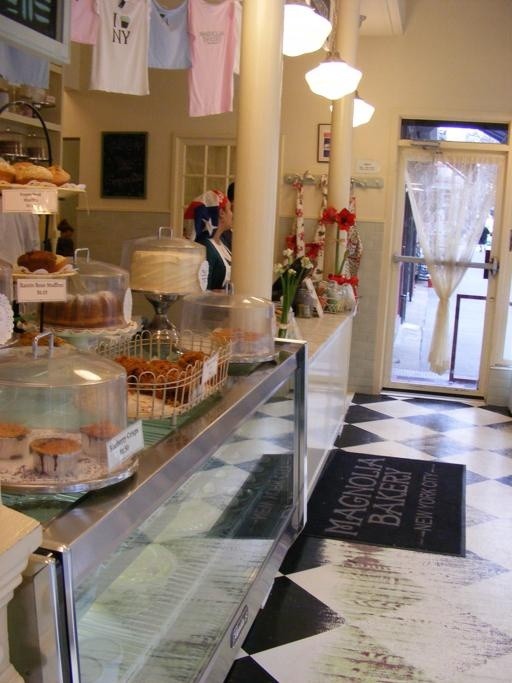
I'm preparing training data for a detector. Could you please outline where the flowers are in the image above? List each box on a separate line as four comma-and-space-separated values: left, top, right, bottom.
318, 207, 359, 284
275, 248, 313, 338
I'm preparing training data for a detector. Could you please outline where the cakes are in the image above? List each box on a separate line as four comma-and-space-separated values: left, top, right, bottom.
130, 250, 205, 293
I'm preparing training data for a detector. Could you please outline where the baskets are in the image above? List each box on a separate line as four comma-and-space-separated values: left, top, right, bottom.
93, 329, 232, 419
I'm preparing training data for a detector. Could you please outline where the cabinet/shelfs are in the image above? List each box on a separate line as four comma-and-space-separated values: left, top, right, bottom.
1, 309, 356, 677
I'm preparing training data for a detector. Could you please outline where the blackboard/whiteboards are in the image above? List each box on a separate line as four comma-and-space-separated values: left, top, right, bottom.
101, 131, 146, 198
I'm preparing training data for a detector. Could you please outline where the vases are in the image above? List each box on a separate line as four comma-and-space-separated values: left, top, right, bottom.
329, 273, 358, 304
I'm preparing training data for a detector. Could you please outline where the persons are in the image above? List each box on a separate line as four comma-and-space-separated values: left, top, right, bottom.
56, 218, 75, 256
183, 188, 232, 291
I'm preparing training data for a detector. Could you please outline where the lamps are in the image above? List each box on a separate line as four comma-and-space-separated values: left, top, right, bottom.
281, 3, 332, 57
317, 122, 332, 162
305, 48, 375, 130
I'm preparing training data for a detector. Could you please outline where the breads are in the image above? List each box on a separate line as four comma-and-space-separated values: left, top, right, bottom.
114, 328, 261, 404
0, 157, 71, 187
43, 290, 118, 326
0, 422, 32, 460
29, 437, 83, 475
80, 420, 122, 458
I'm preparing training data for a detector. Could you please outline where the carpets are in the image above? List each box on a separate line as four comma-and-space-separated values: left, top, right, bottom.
300, 450, 468, 560
